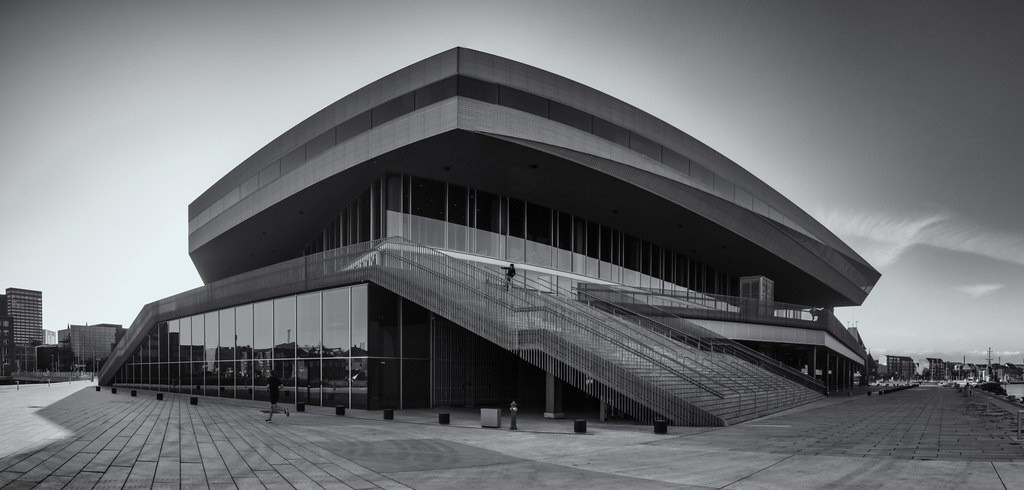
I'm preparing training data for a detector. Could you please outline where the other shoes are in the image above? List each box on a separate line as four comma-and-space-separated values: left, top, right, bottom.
284, 408, 289, 417
265, 419, 272, 423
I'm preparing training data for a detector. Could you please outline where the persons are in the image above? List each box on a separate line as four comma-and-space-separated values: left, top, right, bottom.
501, 264, 515, 291
266, 370, 289, 423
